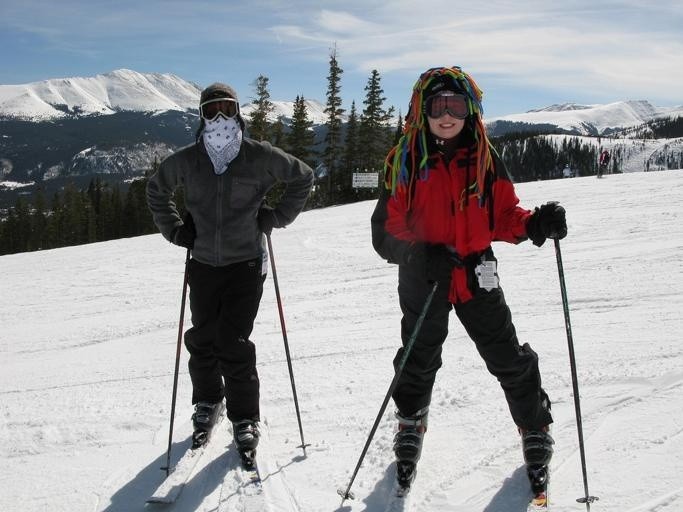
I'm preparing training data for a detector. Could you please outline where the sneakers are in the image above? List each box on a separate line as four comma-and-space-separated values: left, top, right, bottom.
393, 416, 428, 462
519, 426, 556, 471
233, 419, 261, 450
191, 400, 225, 433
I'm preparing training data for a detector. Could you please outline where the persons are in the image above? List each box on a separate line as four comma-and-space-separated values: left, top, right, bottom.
370, 64, 568, 469
561, 164, 571, 178
146, 82, 313, 452
596, 148, 610, 177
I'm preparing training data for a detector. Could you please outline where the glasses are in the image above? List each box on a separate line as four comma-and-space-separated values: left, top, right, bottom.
421, 93, 470, 119
199, 97, 239, 122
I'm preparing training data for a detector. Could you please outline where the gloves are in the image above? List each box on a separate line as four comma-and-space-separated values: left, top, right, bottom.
258, 205, 279, 235
526, 200, 567, 248
170, 224, 194, 251
407, 240, 454, 287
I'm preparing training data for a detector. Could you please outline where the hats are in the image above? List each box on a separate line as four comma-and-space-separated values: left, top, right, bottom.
199, 82, 238, 105
423, 73, 469, 102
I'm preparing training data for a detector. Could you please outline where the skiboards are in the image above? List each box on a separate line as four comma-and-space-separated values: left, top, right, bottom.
145, 405, 270, 511
384, 490, 551, 511
